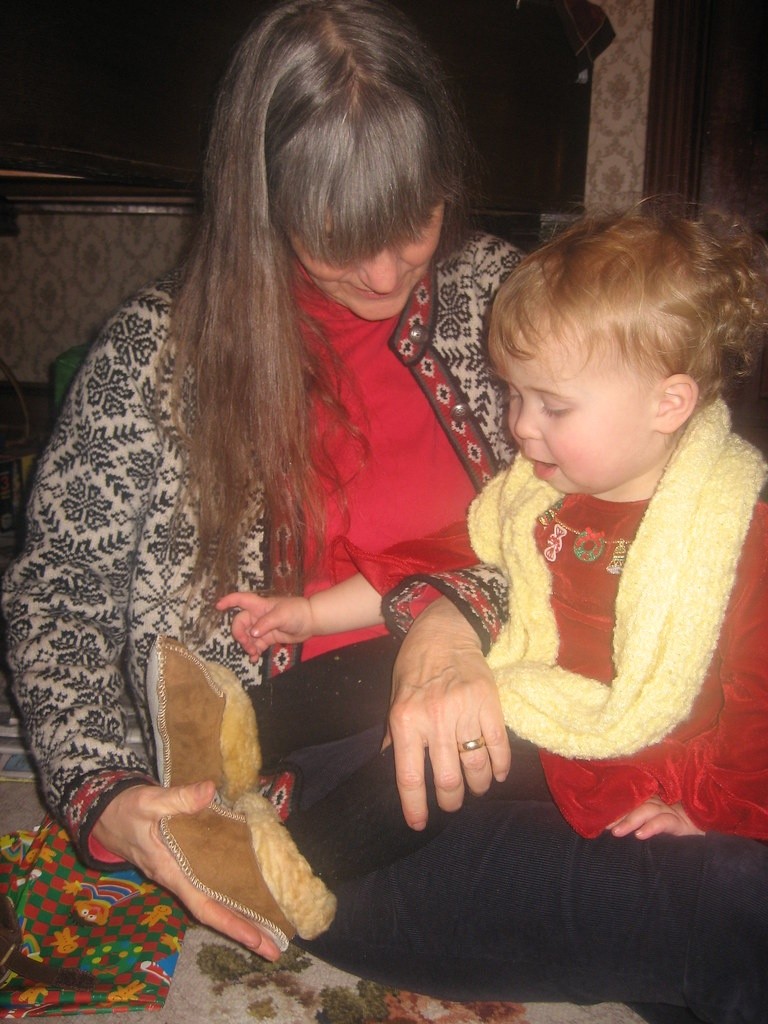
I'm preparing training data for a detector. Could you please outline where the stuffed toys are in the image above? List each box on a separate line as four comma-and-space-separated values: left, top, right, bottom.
144, 638, 335, 953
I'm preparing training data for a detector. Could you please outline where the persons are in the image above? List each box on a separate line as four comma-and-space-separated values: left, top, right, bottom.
218, 199, 767, 843
0, 0, 768, 1024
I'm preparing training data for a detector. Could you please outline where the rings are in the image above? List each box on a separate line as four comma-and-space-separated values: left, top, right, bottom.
457, 736, 485, 753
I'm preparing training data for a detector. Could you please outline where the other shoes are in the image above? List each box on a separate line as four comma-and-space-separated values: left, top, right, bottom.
144, 634, 339, 953
